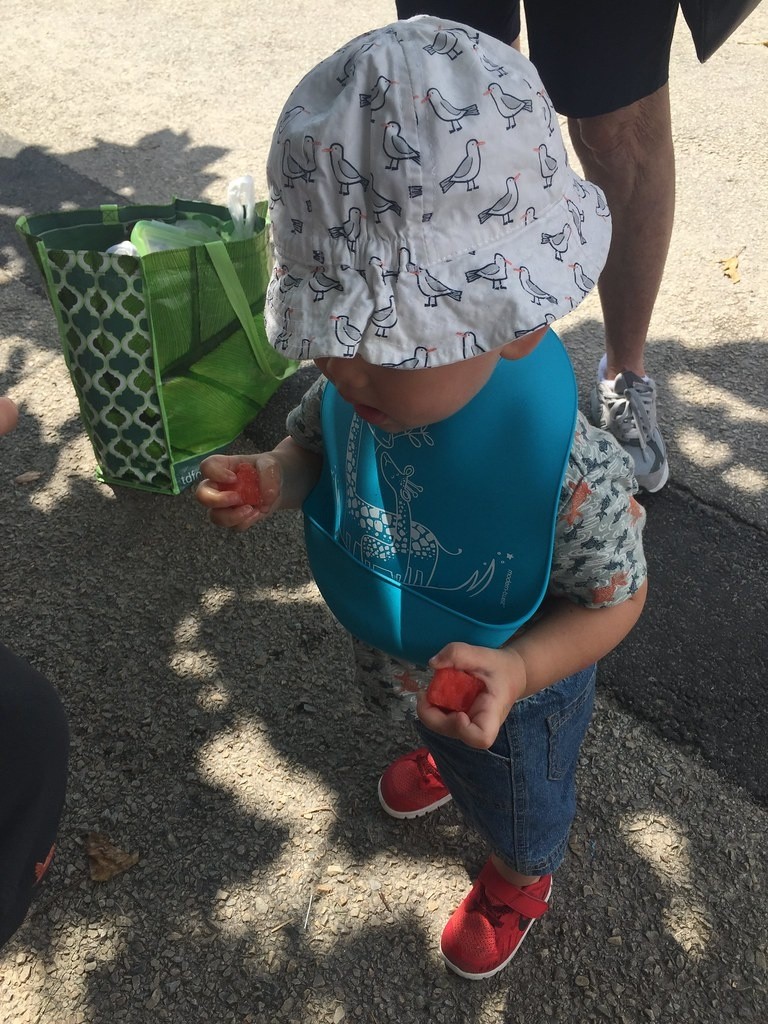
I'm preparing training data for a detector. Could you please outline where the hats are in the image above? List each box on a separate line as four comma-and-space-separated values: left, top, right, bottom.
264, 13, 613, 369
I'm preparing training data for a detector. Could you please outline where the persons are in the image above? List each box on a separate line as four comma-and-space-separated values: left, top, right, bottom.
393, 0, 761, 500
199, 12, 651, 981
0, 651, 70, 953
0, 396, 18, 436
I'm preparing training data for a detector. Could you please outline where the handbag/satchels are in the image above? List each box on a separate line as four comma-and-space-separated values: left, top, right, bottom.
15, 195, 300, 495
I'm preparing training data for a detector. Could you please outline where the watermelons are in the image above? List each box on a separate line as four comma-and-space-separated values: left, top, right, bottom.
428, 668, 484, 712
218, 463, 263, 508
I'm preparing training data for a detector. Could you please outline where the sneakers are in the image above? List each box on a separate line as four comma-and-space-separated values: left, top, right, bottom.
590, 352, 668, 494
439, 857, 553, 980
378, 747, 452, 819
